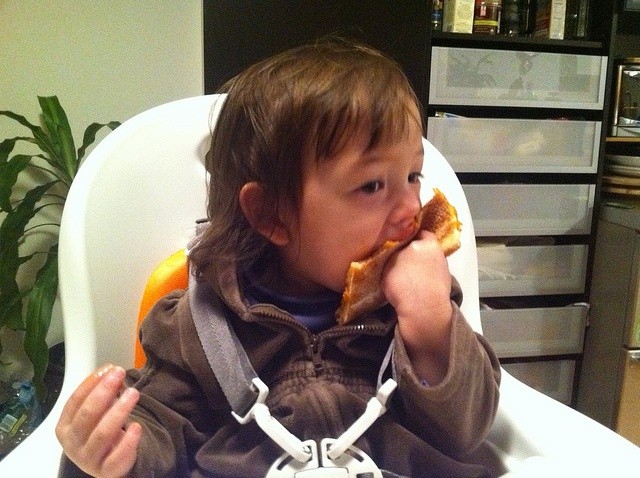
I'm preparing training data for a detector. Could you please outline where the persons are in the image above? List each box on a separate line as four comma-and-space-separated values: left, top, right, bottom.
54, 24, 503, 478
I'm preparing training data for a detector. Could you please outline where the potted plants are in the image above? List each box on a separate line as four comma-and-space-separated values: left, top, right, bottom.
0, 94, 122, 417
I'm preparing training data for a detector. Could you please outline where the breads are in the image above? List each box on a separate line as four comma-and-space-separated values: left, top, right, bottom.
336, 190, 462, 327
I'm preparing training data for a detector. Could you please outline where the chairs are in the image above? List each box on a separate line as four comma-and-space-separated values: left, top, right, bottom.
0, 93, 639, 476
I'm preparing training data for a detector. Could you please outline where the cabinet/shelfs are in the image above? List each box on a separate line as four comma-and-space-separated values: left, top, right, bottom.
410, 1, 619, 409
